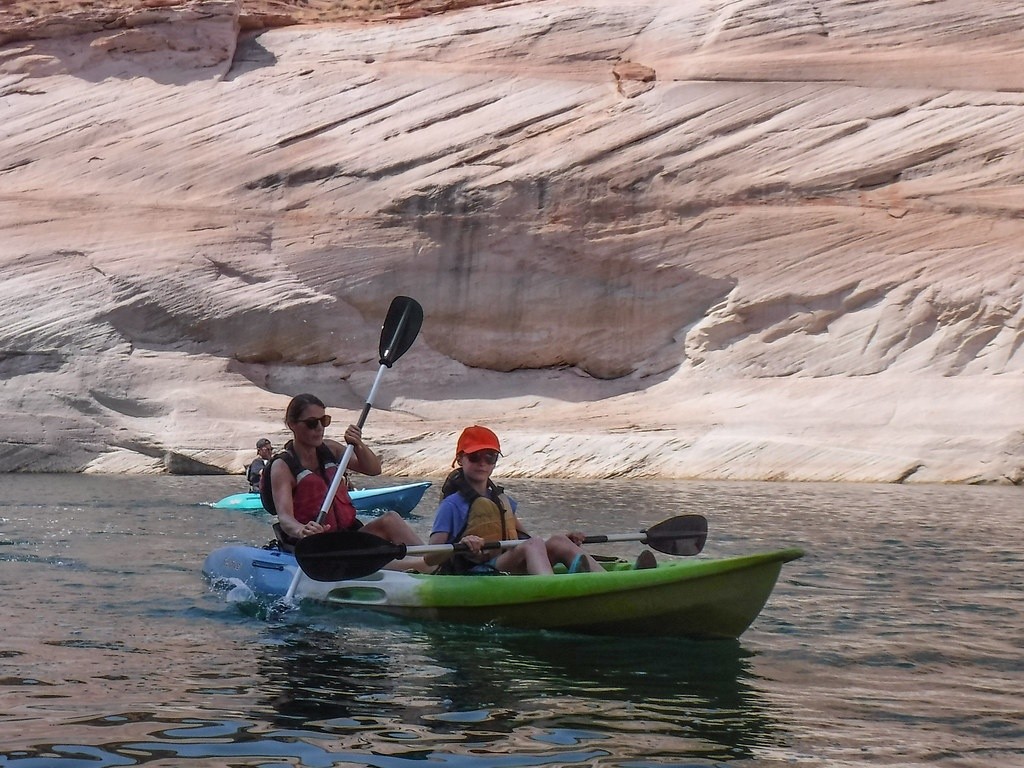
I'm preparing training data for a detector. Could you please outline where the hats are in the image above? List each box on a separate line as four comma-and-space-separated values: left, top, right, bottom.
256, 438, 271, 455
456, 425, 500, 454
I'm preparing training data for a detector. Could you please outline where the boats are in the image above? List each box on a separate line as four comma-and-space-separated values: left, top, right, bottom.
200, 542, 806, 641
209, 480, 433, 517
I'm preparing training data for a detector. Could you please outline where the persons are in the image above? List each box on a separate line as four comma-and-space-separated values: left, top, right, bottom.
424, 426, 658, 575
257, 393, 442, 574
246, 438, 274, 494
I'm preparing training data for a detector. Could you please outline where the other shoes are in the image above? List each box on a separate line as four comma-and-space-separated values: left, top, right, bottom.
568, 553, 590, 573
633, 550, 657, 569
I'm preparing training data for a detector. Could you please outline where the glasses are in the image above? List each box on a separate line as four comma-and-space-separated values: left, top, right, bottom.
462, 451, 498, 464
260, 447, 273, 451
295, 415, 331, 430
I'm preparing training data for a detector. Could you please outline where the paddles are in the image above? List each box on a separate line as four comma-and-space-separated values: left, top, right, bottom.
278, 295, 425, 611
293, 514, 710, 583
